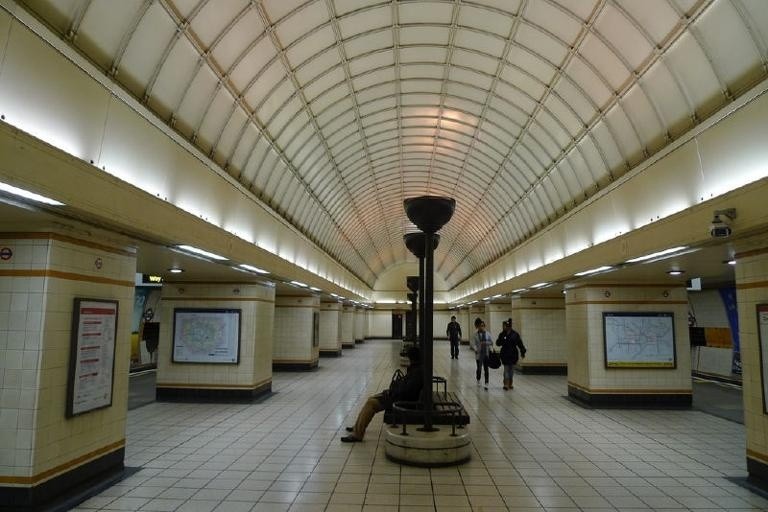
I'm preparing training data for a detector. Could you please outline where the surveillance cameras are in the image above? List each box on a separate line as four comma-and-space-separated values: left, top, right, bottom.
709, 222, 733, 238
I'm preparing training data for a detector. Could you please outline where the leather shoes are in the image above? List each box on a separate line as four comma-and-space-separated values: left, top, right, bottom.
341, 435, 363, 442
346, 426, 365, 433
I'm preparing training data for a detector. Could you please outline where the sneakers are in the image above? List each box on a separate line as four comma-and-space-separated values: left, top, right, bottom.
484, 383, 488, 390
477, 379, 481, 387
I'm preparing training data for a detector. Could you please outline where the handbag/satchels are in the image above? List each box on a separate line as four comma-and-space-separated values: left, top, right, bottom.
388, 368, 409, 395
487, 341, 501, 369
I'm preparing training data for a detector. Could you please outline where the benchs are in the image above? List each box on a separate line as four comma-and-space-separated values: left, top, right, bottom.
383, 370, 470, 436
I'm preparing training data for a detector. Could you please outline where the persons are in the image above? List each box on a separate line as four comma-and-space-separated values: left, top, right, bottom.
469, 320, 495, 390
496, 321, 527, 390
445, 315, 463, 359
340, 346, 422, 443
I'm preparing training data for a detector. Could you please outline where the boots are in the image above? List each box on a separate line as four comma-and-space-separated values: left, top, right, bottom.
502, 378, 510, 390
509, 379, 513, 389
